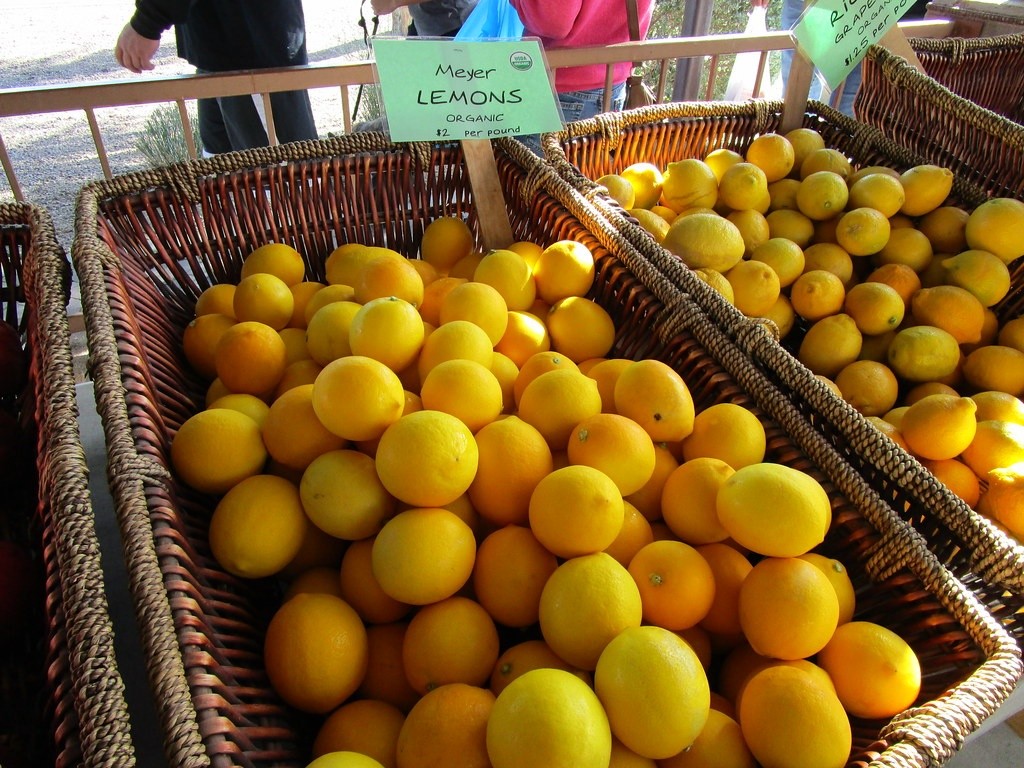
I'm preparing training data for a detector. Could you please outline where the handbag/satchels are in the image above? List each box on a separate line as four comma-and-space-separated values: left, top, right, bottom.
622, 0, 656, 111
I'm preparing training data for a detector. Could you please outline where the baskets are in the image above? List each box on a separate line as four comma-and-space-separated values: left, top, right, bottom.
0, 201, 138, 768
540, 98, 1024, 661
70, 131, 1024, 768
852, 32, 1024, 203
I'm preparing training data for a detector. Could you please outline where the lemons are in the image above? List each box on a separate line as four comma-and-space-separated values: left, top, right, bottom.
170, 218, 920, 768
594, 127, 1024, 548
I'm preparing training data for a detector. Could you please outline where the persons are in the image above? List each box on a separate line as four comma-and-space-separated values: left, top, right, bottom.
748, 1, 870, 121
115, 0, 318, 160
507, 0, 654, 122
370, 0, 482, 40
